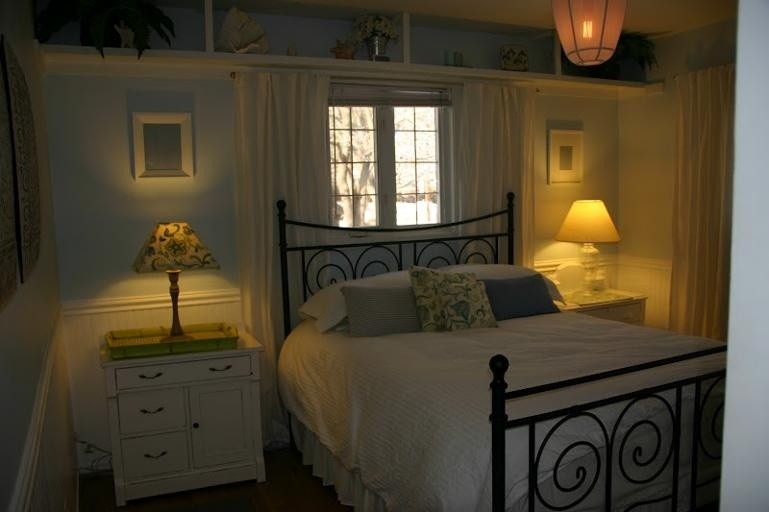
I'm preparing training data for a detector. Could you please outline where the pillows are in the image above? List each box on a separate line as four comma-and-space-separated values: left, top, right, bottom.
409, 265, 498, 332
435, 263, 568, 306
340, 287, 422, 338
297, 269, 411, 333
476, 273, 560, 321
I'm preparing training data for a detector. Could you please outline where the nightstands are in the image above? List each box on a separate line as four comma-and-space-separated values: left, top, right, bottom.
554, 285, 648, 327
100, 321, 266, 508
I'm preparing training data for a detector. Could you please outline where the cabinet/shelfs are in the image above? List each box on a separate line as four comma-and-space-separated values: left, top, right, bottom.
29, 0, 646, 88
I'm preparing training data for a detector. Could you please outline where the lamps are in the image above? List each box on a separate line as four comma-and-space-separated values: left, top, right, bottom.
552, 0, 627, 67
130, 220, 221, 343
555, 199, 634, 306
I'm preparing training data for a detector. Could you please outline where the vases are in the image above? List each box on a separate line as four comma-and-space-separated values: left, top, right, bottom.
365, 35, 391, 62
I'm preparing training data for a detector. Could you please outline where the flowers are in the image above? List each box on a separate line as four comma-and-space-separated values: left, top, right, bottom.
352, 14, 402, 40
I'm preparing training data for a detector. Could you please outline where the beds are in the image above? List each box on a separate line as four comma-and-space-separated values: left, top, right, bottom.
277, 192, 726, 511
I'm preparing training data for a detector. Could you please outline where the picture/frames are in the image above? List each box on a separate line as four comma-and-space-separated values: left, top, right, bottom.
547, 128, 584, 185
132, 112, 194, 181
